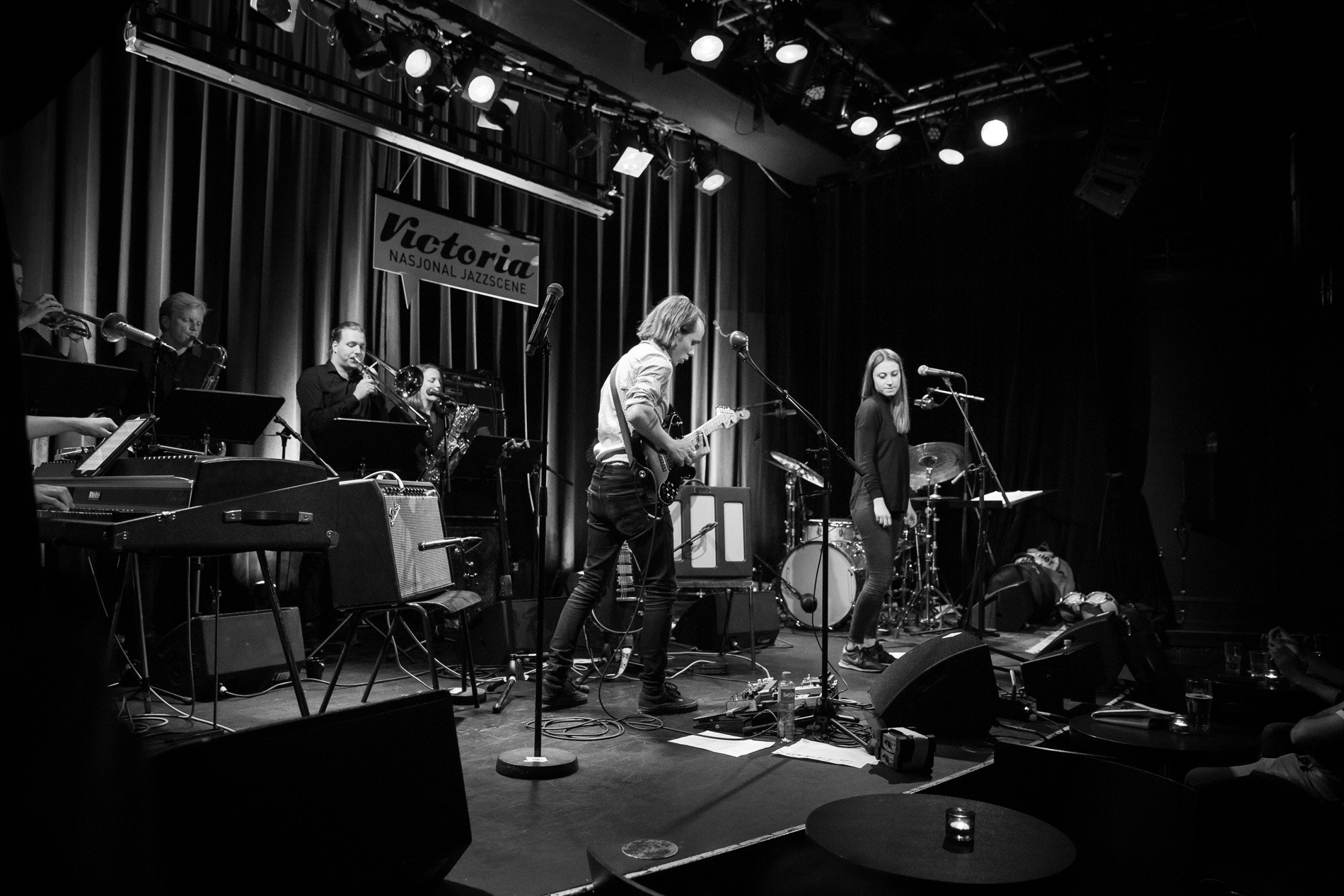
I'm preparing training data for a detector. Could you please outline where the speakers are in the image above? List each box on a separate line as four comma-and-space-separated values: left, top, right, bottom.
189, 606, 307, 703
1, 690, 473, 896
867, 629, 997, 743
1018, 600, 1158, 714
325, 476, 457, 611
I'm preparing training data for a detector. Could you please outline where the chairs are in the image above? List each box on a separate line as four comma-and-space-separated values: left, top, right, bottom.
999, 741, 1221, 896
316, 590, 479, 713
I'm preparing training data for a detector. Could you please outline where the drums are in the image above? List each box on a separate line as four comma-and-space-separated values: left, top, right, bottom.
780, 515, 869, 629
1020, 548, 1128, 620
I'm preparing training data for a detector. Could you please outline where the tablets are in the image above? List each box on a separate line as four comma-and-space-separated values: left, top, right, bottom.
69, 410, 158, 479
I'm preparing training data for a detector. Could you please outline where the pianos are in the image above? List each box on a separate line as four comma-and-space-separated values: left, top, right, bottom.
21, 441, 340, 733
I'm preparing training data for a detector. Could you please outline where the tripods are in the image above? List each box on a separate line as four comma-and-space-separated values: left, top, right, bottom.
485, 468, 590, 714
114, 552, 195, 713
741, 451, 878, 754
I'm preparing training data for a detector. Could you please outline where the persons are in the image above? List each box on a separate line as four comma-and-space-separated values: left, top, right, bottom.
838, 348, 918, 673
26, 413, 119, 511
123, 293, 230, 686
1184, 627, 1344, 896
543, 294, 711, 714
11, 250, 89, 362
296, 321, 449, 654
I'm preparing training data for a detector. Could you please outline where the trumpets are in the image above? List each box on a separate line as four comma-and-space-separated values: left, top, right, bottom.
27, 301, 126, 345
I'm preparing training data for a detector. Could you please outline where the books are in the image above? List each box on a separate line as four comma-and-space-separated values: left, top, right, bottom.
71, 414, 161, 477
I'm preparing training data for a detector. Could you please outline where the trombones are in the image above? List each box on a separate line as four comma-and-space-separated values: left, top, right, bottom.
351, 352, 435, 441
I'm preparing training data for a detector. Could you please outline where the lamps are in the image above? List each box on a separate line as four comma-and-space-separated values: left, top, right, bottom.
247, 1, 1024, 196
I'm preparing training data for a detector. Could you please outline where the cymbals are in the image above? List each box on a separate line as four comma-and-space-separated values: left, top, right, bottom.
899, 439, 975, 489
758, 450, 832, 492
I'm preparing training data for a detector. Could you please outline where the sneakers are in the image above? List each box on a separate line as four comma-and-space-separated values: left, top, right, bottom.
861, 641, 897, 666
541, 678, 589, 707
838, 644, 886, 672
637, 681, 699, 717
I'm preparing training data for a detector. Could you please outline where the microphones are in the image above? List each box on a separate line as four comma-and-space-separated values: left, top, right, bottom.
917, 363, 964, 380
522, 280, 565, 359
426, 385, 454, 404
418, 534, 482, 553
711, 318, 731, 338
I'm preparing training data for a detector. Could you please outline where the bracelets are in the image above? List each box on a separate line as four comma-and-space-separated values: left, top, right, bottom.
69, 335, 84, 342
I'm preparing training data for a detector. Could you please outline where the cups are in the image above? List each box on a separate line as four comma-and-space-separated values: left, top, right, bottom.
1224, 641, 1243, 674
946, 807, 975, 841
1260, 633, 1279, 679
1170, 714, 1189, 733
1184, 678, 1212, 731
1249, 650, 1269, 683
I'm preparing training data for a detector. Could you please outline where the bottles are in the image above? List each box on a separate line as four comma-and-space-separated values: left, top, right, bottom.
1061, 639, 1072, 650
778, 671, 796, 739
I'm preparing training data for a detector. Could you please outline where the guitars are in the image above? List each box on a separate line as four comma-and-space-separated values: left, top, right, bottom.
587, 537, 651, 636
632, 406, 752, 508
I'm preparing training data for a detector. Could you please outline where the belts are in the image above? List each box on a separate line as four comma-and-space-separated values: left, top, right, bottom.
594, 461, 632, 476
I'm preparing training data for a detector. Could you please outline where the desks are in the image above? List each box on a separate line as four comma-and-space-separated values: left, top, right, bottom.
802, 791, 1077, 885
1192, 662, 1289, 690
1071, 712, 1228, 753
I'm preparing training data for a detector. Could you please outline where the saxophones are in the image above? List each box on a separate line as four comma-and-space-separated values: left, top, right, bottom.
190, 335, 227, 457
417, 385, 479, 490
